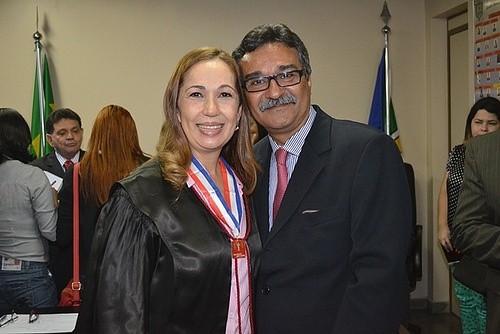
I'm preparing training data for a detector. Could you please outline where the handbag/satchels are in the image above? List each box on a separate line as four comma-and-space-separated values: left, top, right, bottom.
59, 279, 80, 307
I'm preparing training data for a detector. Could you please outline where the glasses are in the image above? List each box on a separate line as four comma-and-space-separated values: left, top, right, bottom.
28, 308, 39, 323
241, 69, 304, 93
0, 312, 18, 327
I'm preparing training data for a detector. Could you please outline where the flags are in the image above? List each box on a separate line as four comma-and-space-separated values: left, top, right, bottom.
30, 51, 55, 159
368, 48, 402, 154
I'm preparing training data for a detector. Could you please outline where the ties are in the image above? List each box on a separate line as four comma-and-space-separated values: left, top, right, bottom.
273, 147, 288, 225
62, 161, 74, 172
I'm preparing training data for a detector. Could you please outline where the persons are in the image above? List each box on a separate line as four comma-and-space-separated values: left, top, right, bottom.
437, 96, 500, 334
28, 107, 87, 179
230, 24, 411, 334
0, 108, 64, 310
56, 105, 152, 288
73, 47, 259, 334
